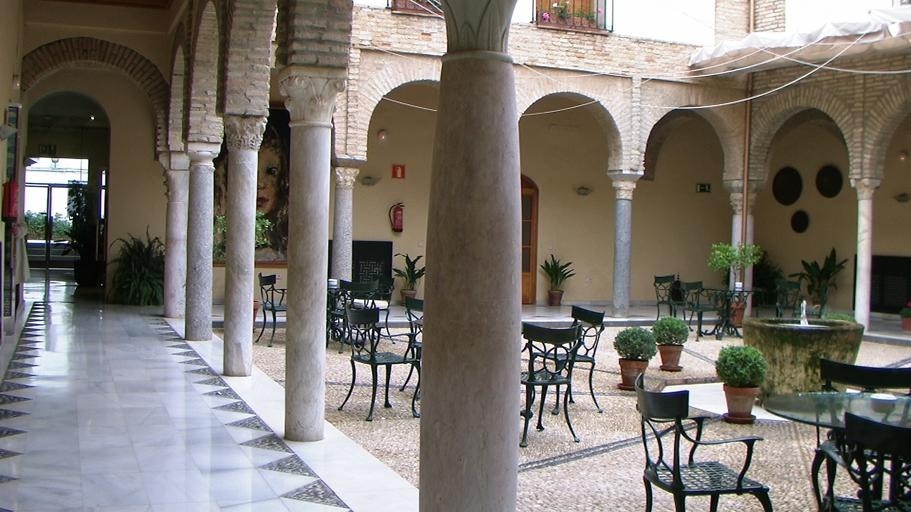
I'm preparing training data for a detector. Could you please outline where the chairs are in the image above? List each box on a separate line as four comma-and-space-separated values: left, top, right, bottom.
257, 272, 394, 355
337, 306, 608, 448
653, 274, 822, 342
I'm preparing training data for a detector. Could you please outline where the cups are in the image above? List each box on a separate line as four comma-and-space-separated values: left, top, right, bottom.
735, 282, 743, 291
329, 279, 337, 286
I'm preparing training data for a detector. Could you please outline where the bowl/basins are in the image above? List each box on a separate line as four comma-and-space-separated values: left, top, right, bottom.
870, 393, 897, 413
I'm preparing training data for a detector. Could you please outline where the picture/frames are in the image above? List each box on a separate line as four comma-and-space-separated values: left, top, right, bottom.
213, 107, 289, 268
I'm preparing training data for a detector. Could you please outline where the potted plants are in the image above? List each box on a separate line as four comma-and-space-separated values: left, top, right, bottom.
715, 345, 768, 423
392, 251, 425, 307
706, 242, 764, 328
539, 254, 576, 305
104, 222, 166, 305
63, 183, 103, 287
613, 315, 689, 391
788, 246, 849, 312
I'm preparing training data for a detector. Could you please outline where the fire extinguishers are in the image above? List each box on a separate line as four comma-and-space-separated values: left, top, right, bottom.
389, 203, 406, 232
2, 179, 18, 222
